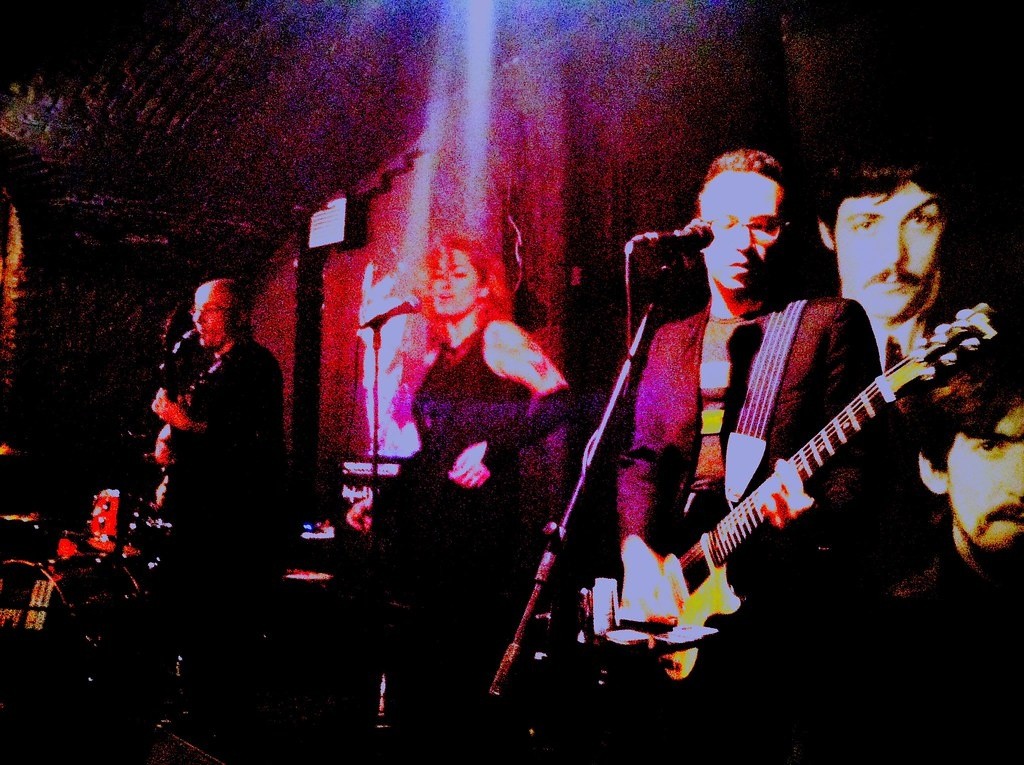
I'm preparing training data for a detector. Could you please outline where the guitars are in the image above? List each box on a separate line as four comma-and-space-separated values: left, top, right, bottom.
635, 300, 1000, 679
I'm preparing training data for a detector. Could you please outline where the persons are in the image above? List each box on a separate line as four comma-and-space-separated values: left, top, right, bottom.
812, 151, 1024, 632
151, 279, 284, 607
346, 232, 570, 764
616, 149, 895, 631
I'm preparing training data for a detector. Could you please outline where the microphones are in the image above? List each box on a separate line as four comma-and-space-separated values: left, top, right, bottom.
633, 218, 715, 253
358, 296, 424, 329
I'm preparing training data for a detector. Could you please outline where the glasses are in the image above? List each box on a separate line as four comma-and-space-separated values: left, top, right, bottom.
188, 304, 234, 316
